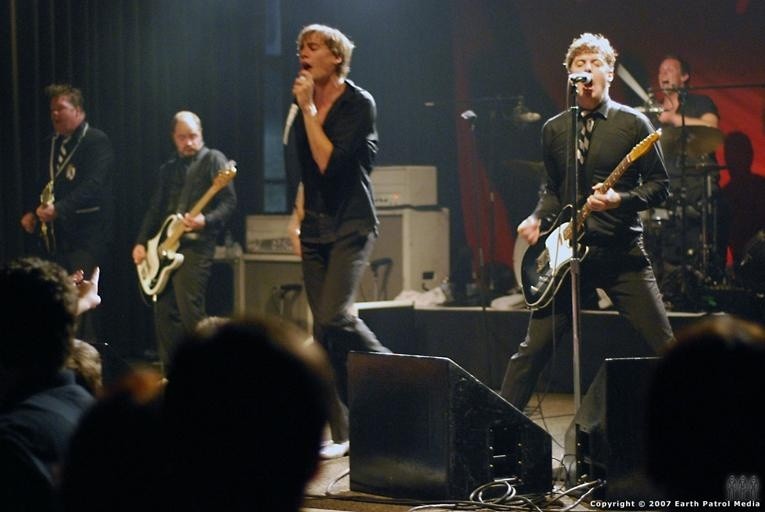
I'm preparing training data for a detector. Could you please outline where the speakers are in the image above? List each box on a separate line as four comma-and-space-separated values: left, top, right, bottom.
346, 349, 552, 500
352, 206, 450, 305
563, 355, 661, 505
203, 255, 313, 347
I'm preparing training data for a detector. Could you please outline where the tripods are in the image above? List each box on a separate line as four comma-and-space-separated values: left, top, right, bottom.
643, 84, 719, 313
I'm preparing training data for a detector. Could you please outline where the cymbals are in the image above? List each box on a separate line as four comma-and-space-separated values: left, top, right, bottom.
661, 124, 721, 162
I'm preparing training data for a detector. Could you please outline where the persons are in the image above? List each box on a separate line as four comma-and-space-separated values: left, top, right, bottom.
284, 22, 394, 460
638, 55, 724, 286
20, 83, 115, 284
641, 313, 764, 512
132, 110, 238, 378
499, 31, 678, 413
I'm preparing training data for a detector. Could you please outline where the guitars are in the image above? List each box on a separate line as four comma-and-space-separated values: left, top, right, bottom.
36, 179, 57, 262
522, 126, 661, 312
134, 160, 237, 296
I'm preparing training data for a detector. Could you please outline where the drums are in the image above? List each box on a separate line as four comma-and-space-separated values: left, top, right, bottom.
639, 200, 707, 233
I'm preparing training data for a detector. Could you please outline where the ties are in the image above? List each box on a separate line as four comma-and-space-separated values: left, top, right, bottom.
54, 139, 70, 173
574, 113, 595, 175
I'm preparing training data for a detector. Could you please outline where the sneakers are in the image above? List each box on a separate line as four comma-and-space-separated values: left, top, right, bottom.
319, 435, 350, 460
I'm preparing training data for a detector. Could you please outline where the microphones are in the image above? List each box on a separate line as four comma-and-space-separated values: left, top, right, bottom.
570, 73, 592, 84
285, 96, 299, 126
